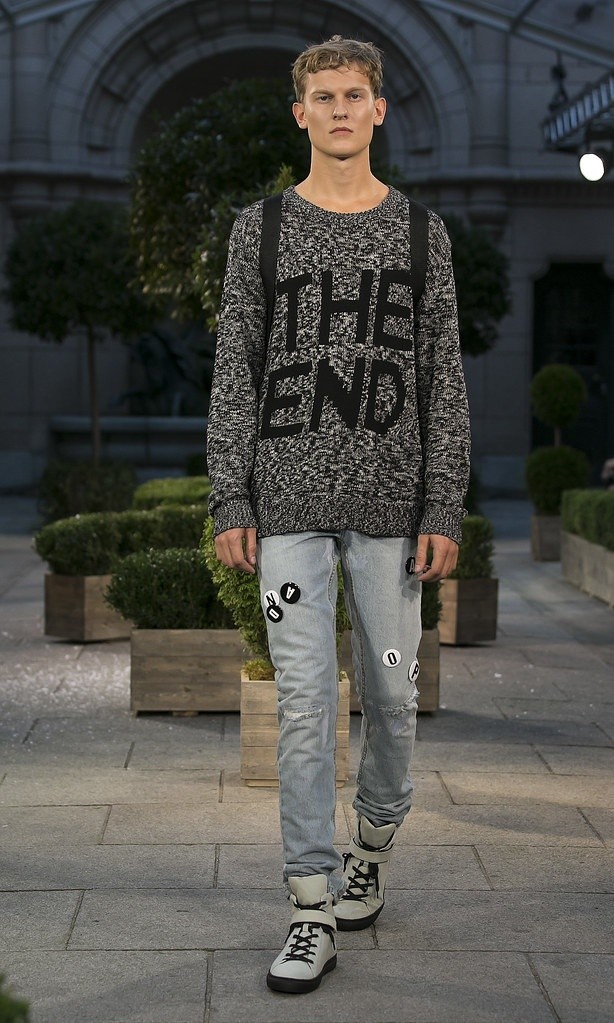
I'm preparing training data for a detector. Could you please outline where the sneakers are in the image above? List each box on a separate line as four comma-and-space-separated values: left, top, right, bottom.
333, 817, 399, 931
267, 876, 340, 993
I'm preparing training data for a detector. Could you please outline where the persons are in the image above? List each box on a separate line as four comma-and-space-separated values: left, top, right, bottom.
207, 36, 473, 996
